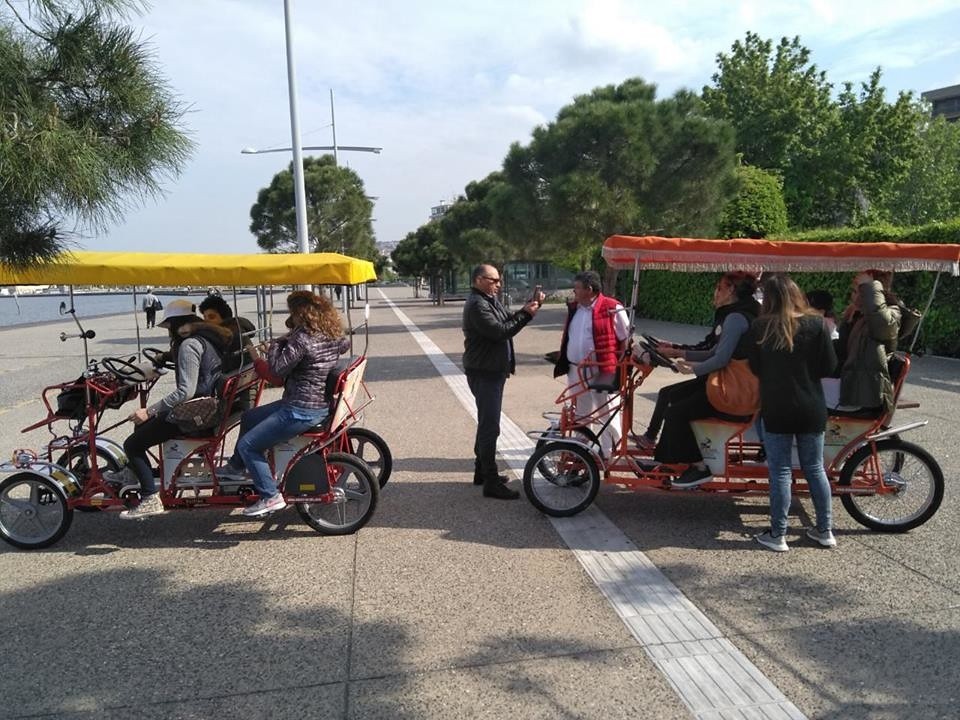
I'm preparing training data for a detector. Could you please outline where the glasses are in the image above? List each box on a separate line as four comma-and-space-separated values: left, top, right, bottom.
480, 276, 500, 284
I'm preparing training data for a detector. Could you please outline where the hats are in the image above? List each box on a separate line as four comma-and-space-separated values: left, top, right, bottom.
155, 299, 196, 328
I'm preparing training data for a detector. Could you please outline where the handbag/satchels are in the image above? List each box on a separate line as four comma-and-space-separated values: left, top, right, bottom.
174, 394, 220, 434
152, 299, 163, 310
706, 358, 763, 416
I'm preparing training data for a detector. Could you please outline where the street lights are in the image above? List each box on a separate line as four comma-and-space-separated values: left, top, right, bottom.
241, 87, 383, 315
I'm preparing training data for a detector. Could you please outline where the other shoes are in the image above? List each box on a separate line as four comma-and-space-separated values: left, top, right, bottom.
474, 473, 509, 485
480, 471, 520, 499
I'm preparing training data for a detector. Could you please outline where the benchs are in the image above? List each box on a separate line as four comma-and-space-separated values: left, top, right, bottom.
826, 354, 912, 471
270, 354, 368, 472
692, 406, 760, 476
162, 361, 267, 491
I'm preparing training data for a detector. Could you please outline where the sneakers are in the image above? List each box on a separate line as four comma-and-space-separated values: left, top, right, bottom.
214, 463, 246, 481
635, 461, 658, 479
756, 527, 789, 551
671, 464, 713, 487
102, 465, 138, 487
806, 527, 837, 546
626, 433, 656, 449
243, 492, 286, 516
119, 491, 164, 520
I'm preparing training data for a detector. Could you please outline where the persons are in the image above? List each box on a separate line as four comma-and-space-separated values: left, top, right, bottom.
747, 277, 836, 552
334, 286, 341, 300
103, 295, 260, 498
218, 290, 352, 516
554, 272, 632, 470
208, 287, 222, 299
143, 289, 158, 329
637, 269, 901, 489
462, 265, 546, 498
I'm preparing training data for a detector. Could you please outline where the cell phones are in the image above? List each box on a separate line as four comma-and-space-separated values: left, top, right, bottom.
532, 285, 543, 301
